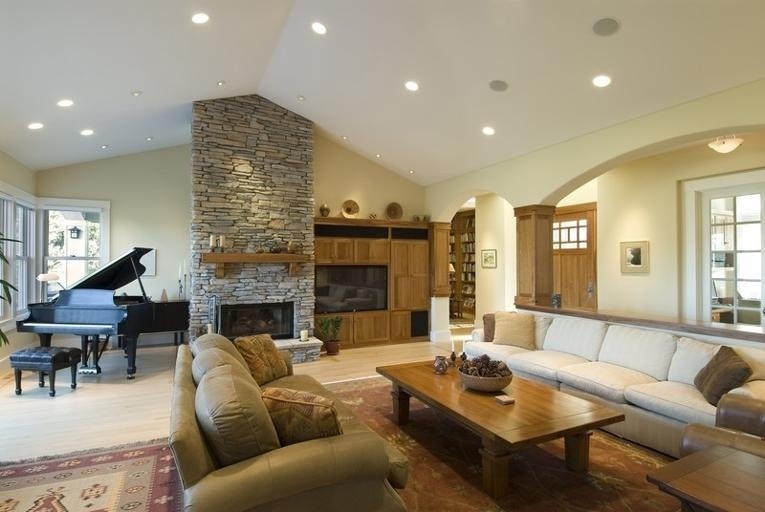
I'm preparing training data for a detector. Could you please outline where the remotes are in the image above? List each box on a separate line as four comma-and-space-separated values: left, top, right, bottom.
496, 395, 514, 404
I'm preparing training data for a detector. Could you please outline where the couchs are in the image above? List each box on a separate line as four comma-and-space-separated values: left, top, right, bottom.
453, 305, 764, 461
167, 329, 412, 510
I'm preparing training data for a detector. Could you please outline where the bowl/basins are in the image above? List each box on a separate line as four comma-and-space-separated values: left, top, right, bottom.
412, 215, 431, 222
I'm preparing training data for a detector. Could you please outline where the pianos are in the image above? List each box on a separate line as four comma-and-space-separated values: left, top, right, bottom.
16, 247, 189, 380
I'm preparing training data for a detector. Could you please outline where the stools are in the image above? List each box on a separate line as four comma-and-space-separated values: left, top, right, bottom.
450, 299, 464, 318
8, 343, 80, 398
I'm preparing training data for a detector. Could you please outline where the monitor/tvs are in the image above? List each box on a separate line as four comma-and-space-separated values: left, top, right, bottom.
315, 264, 388, 314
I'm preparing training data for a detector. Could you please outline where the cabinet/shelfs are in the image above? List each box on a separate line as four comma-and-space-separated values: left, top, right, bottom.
353, 310, 390, 349
313, 238, 353, 265
313, 313, 353, 351
388, 239, 429, 311
714, 215, 735, 253
354, 239, 391, 266
448, 210, 475, 318
388, 310, 412, 346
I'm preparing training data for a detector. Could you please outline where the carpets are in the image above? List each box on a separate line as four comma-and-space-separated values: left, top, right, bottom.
301, 366, 691, 512
0, 438, 184, 512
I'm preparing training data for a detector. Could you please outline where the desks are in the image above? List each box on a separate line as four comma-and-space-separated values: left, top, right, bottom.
644, 443, 764, 512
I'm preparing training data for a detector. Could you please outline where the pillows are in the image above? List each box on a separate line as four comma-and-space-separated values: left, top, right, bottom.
229, 331, 290, 383
256, 384, 342, 442
482, 311, 495, 342
693, 346, 752, 407
490, 309, 539, 352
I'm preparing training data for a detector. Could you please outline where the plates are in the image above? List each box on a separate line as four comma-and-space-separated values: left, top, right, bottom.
341, 200, 360, 219
385, 202, 404, 221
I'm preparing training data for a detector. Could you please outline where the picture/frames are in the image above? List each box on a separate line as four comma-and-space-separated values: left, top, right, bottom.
619, 240, 650, 274
481, 248, 497, 268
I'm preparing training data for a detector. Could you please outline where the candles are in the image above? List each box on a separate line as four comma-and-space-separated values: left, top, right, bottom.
207, 322, 212, 333
178, 262, 182, 280
299, 329, 309, 340
183, 257, 186, 273
209, 234, 217, 247
219, 235, 225, 248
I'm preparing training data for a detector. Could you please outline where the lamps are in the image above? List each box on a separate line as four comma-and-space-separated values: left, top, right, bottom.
449, 263, 455, 298
68, 227, 81, 239
35, 272, 66, 291
707, 131, 749, 155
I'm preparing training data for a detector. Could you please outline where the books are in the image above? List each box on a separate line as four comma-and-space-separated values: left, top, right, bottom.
450, 232, 475, 297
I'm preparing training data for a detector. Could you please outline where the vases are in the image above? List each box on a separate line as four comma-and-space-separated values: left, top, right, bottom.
159, 288, 168, 303
319, 203, 331, 217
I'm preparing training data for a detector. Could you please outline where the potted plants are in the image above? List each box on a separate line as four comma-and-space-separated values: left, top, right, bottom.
319, 317, 343, 354
